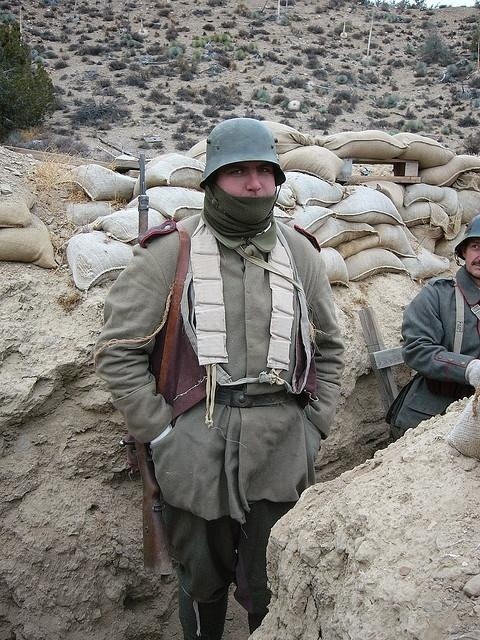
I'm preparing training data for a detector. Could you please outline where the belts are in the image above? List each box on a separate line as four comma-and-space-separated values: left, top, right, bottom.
214, 386, 292, 408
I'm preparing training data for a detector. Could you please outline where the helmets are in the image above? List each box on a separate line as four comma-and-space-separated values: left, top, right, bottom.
454, 214, 480, 260
198, 116, 286, 188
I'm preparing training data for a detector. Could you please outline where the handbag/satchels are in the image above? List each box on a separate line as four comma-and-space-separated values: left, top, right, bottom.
382, 371, 475, 442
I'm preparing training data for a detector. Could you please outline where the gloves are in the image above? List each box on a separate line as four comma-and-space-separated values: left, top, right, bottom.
465, 358, 480, 391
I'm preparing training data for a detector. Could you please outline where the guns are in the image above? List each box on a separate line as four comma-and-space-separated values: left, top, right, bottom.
119, 155, 174, 576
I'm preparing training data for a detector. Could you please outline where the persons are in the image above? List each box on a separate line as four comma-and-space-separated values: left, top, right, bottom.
93, 118, 348, 640
386, 215, 480, 442
